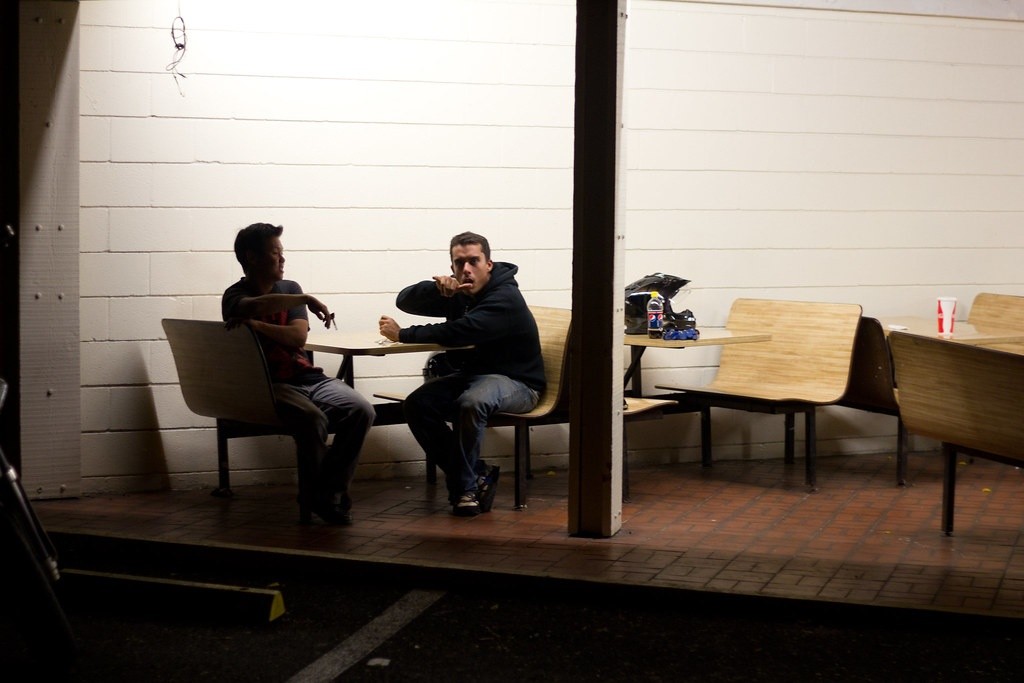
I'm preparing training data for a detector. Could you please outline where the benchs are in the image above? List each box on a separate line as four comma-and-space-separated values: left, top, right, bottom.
888, 330, 1024, 533
655, 298, 862, 490
621, 397, 677, 502
783, 316, 906, 480
162, 317, 324, 529
372, 305, 572, 512
970, 293, 1024, 332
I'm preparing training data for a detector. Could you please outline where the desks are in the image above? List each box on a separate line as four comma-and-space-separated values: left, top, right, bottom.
304, 327, 473, 391
875, 315, 1023, 344
623, 327, 772, 396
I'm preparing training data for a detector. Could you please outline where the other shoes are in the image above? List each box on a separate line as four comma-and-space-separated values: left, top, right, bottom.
453, 492, 479, 517
297, 486, 352, 525
478, 465, 501, 512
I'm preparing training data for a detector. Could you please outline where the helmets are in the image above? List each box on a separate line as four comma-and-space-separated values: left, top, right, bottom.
625, 273, 696, 335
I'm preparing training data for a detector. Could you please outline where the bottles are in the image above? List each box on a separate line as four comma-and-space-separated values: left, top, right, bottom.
646, 290, 664, 339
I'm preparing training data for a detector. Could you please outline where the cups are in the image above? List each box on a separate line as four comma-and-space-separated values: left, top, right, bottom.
937, 297, 958, 337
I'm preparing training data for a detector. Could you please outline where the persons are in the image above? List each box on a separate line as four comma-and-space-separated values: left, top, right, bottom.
378, 231, 549, 519
219, 223, 376, 530
